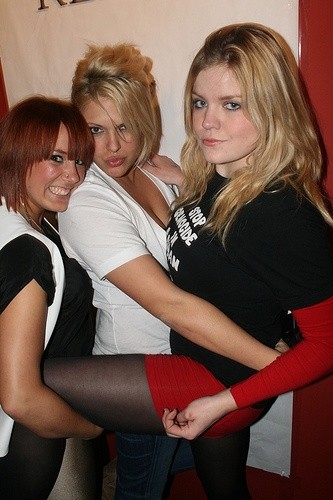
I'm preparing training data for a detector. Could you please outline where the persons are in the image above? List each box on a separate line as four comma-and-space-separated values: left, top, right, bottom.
1, 97, 104, 500
55, 47, 293, 500
3, 22, 333, 500
